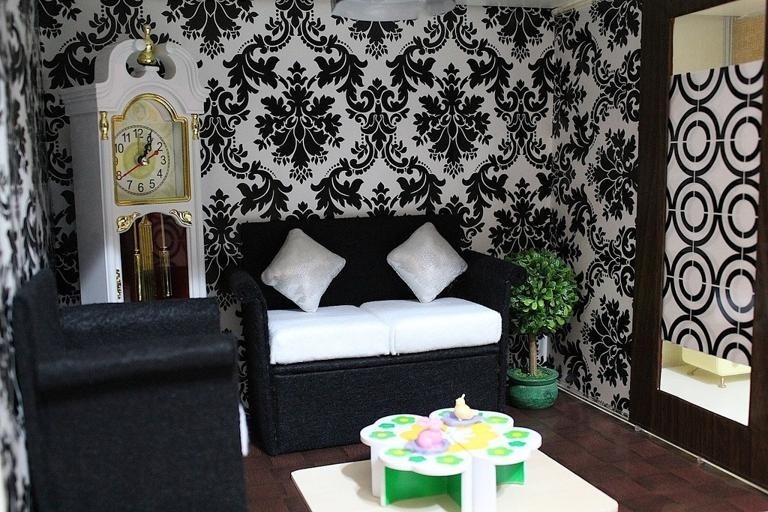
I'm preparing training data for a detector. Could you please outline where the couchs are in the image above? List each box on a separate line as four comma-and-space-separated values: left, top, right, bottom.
13, 266, 247, 511
219, 218, 531, 456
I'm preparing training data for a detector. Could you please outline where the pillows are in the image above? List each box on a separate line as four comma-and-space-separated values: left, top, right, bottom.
385, 220, 470, 304
258, 227, 347, 316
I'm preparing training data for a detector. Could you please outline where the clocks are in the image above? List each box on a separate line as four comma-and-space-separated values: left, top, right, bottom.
60, 24, 211, 305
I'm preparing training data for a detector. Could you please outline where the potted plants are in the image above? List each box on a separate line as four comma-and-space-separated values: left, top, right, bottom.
500, 244, 580, 412
